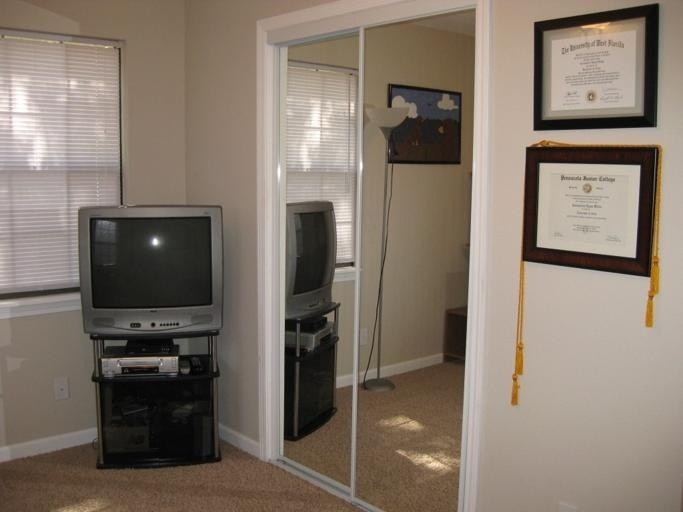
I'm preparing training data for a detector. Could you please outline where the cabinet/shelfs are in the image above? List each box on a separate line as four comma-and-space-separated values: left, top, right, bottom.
284, 301, 341, 443
89, 329, 223, 470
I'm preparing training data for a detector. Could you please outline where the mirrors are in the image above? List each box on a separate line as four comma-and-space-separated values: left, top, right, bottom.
258, 0, 492, 512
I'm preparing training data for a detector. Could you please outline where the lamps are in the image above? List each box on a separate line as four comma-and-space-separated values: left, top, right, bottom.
360, 105, 407, 392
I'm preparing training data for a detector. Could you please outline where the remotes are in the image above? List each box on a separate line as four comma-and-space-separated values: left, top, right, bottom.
180, 361, 190, 374
191, 356, 202, 373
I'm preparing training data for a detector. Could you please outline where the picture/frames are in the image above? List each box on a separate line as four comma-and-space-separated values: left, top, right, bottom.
520, 144, 660, 276
386, 82, 463, 165
532, 2, 658, 130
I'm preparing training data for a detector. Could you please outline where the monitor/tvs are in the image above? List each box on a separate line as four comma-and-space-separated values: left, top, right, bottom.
285, 201, 338, 320
78, 204, 224, 335
